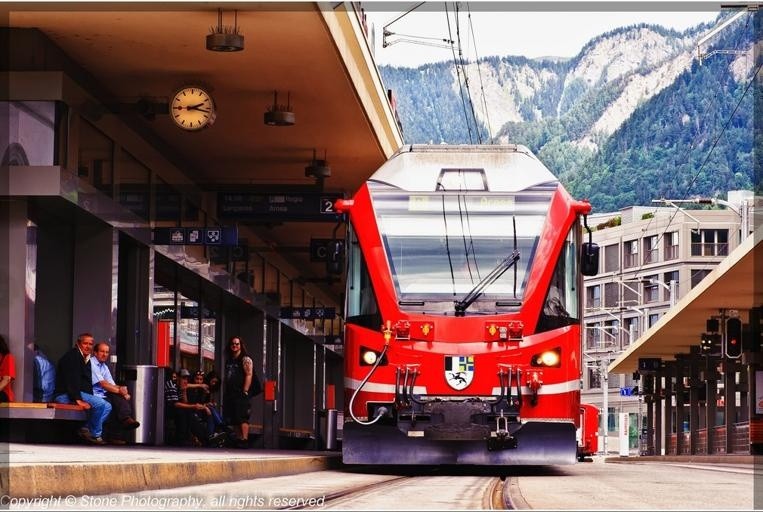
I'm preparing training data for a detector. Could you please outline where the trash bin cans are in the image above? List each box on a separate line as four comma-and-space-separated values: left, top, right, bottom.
123, 364, 164, 446
639, 428, 648, 456
317, 409, 338, 451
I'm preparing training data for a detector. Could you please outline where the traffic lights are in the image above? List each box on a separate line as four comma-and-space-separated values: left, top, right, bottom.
725, 317, 743, 359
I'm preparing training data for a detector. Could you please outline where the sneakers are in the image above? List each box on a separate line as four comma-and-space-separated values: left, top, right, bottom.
182, 431, 247, 448
121, 417, 140, 431
107, 433, 126, 444
76, 426, 107, 445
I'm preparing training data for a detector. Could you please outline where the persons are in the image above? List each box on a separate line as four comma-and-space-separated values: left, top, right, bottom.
22, 349, 44, 403
0, 334, 14, 402
161, 367, 226, 449
26, 343, 57, 403
218, 335, 253, 449
52, 331, 111, 446
88, 340, 140, 445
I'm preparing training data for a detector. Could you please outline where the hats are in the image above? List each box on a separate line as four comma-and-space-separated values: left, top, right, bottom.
178, 369, 190, 377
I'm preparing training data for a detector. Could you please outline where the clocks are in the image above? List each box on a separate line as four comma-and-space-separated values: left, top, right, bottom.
168, 85, 216, 133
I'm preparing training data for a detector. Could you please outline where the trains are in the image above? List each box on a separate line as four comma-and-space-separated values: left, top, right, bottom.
334, 144, 600, 475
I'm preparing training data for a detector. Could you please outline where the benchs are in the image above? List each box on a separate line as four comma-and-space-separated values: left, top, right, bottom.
247, 423, 310, 439
0, 401, 88, 422
